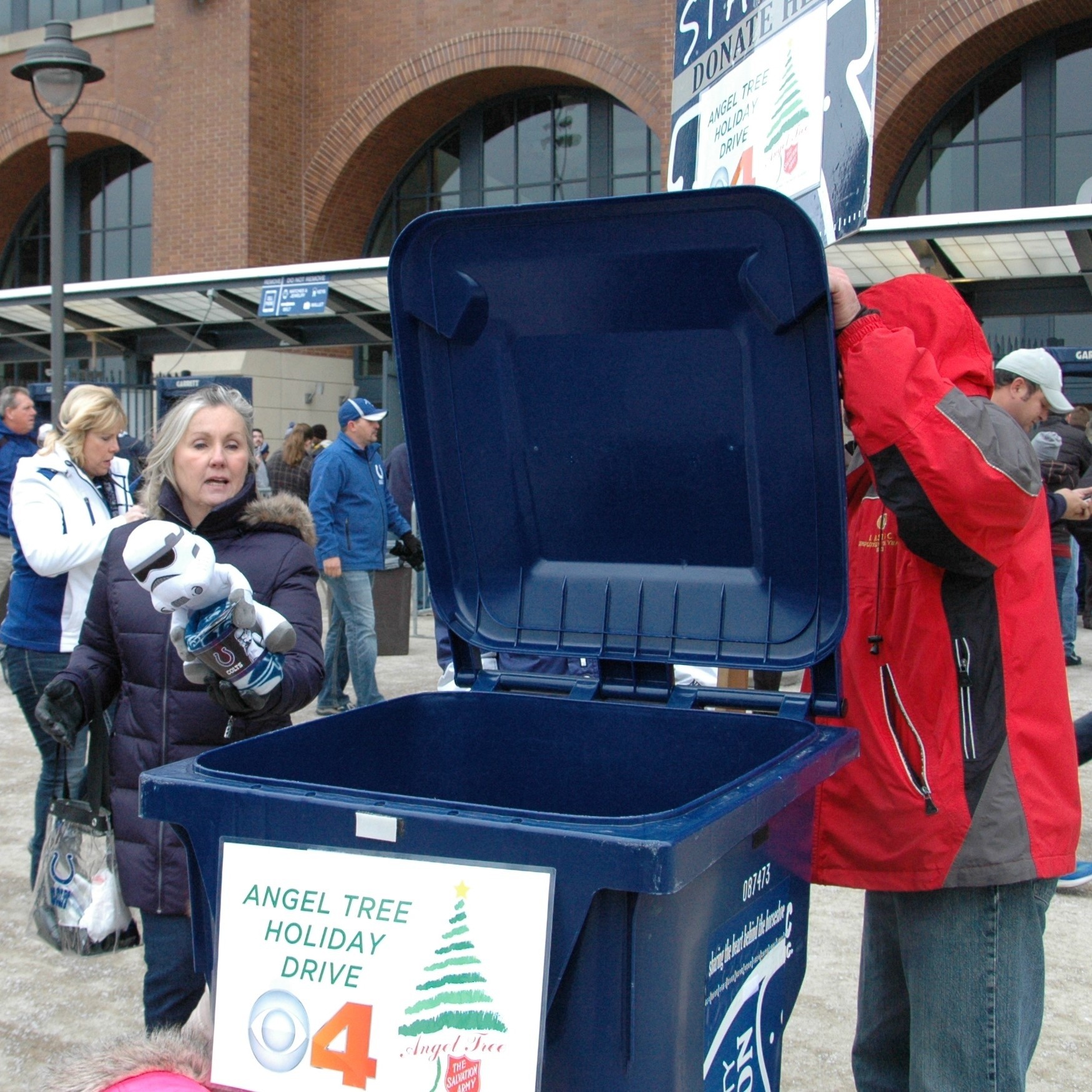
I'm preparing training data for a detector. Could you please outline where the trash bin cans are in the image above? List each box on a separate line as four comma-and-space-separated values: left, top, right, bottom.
136, 186, 862, 1092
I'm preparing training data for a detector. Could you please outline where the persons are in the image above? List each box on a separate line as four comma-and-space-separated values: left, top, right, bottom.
2, 382, 428, 1040
824, 262, 1092, 1092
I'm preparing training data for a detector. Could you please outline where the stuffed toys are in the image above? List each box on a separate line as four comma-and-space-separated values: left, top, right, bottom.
122, 518, 295, 684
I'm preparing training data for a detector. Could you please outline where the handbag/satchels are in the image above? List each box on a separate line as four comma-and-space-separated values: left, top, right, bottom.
26, 670, 140, 957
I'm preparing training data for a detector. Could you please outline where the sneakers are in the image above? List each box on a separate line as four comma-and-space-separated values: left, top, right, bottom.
317, 701, 357, 714
1065, 652, 1082, 665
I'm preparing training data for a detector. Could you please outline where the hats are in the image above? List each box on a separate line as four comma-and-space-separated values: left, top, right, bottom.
284, 422, 295, 439
338, 397, 389, 426
998, 347, 1073, 414
1031, 431, 1062, 463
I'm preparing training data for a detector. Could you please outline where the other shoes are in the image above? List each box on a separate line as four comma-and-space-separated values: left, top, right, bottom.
1056, 861, 1092, 887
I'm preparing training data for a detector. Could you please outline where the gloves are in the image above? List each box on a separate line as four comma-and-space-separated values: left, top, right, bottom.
202, 672, 280, 724
389, 531, 424, 573
35, 678, 86, 750
